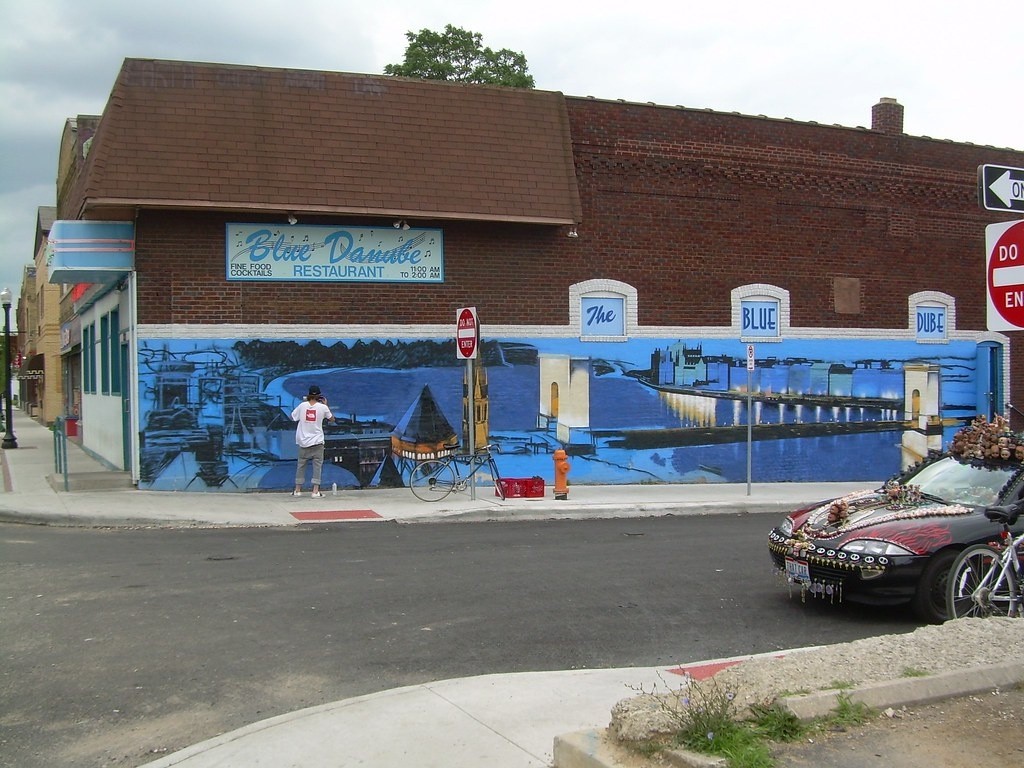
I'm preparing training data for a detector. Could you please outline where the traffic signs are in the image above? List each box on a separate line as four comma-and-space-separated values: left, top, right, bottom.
977, 164, 1024, 213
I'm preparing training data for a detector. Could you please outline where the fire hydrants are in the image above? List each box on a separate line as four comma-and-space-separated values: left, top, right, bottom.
551, 447, 570, 500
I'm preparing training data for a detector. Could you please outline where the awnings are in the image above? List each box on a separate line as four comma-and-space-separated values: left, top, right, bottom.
17, 354, 44, 380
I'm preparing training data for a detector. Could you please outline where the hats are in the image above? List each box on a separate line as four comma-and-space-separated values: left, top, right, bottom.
309, 386, 320, 395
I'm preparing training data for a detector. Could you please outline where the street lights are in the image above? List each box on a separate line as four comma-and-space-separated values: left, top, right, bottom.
0, 287, 20, 449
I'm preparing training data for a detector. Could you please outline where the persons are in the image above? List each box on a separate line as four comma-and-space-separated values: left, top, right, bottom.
289, 385, 335, 498
827, 415, 1024, 523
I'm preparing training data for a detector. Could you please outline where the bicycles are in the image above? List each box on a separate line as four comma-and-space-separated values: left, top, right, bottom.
945, 502, 1024, 620
409, 443, 505, 502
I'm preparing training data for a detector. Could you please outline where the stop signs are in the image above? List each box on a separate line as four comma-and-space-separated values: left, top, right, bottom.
456, 306, 477, 359
985, 219, 1024, 332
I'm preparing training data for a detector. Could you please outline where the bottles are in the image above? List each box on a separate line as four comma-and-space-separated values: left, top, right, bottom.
332, 482, 337, 495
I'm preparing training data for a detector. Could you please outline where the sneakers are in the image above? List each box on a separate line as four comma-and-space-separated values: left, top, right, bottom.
290, 491, 302, 496
311, 492, 326, 498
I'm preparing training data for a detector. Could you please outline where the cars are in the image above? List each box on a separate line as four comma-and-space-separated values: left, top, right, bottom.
769, 450, 1024, 626
